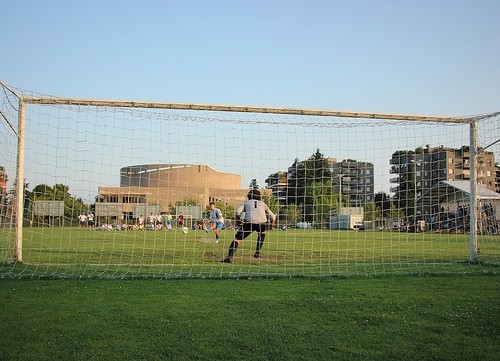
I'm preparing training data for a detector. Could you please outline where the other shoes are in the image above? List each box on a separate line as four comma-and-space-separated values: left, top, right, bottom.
204, 228, 208, 232
254, 253, 261, 258
216, 239, 220, 243
221, 258, 232, 262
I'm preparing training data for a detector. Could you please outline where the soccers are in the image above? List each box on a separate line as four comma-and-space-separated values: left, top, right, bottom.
182, 227, 189, 234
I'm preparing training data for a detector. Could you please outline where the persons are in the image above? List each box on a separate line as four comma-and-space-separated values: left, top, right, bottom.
221, 190, 276, 264
281, 223, 286, 232
394, 203, 499, 233
79, 211, 235, 231
205, 202, 224, 243
